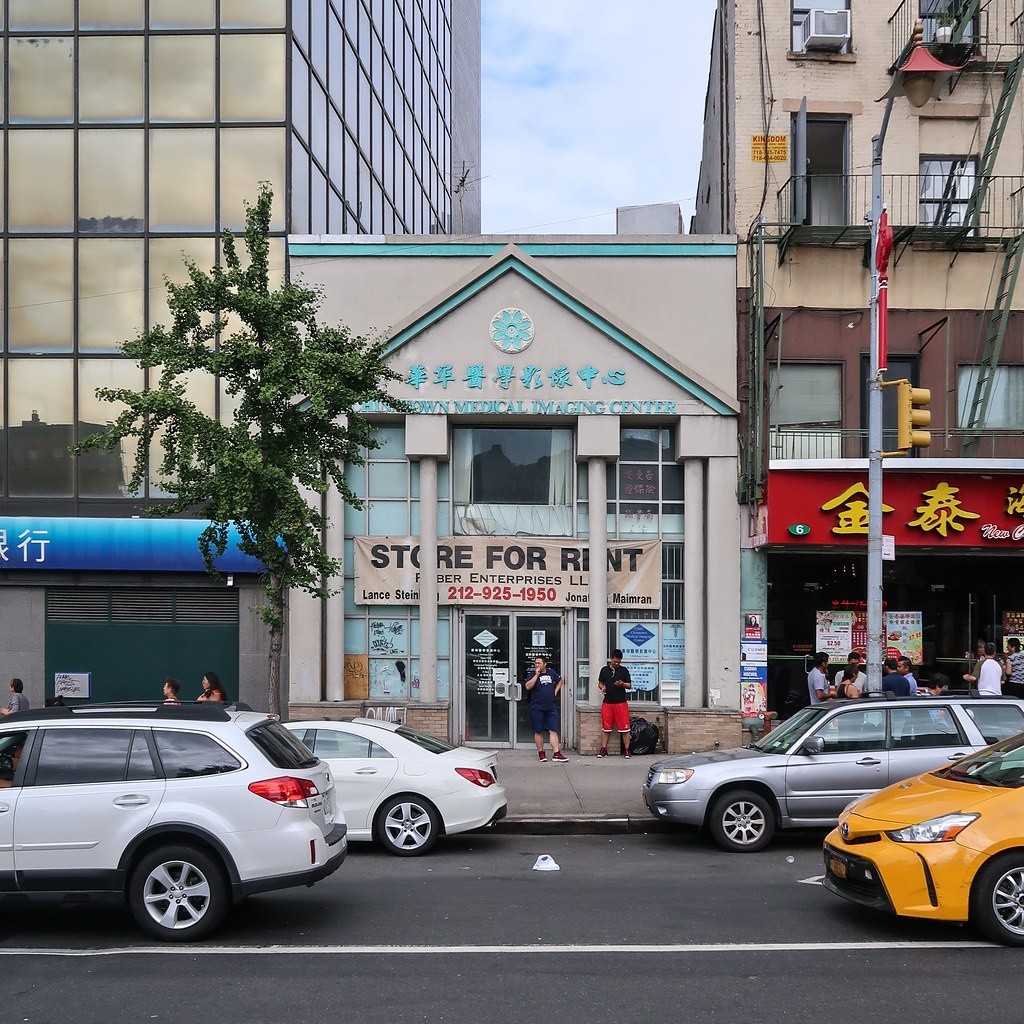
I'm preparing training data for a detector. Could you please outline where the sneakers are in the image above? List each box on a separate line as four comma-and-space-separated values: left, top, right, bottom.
552, 750, 569, 761
539, 750, 547, 761
625, 748, 631, 758
597, 746, 607, 757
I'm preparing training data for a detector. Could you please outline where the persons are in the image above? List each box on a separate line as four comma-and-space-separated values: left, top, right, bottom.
197, 672, 227, 702
896, 656, 917, 695
1004, 638, 1024, 698
882, 658, 910, 697
748, 616, 759, 627
962, 643, 1007, 696
928, 673, 948, 726
524, 656, 569, 762
974, 640, 985, 657
807, 651, 837, 705
835, 652, 868, 692
835, 664, 860, 699
163, 678, 180, 699
0, 679, 29, 717
597, 649, 632, 759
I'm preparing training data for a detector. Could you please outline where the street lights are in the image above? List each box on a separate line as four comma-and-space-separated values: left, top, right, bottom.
861, 18, 970, 697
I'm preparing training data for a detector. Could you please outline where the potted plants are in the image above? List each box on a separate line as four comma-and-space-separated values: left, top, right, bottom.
936, 13, 954, 43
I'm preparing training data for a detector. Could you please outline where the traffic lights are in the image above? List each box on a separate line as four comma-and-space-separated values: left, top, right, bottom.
897, 382, 932, 450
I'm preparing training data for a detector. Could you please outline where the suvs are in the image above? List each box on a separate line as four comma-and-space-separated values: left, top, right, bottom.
0, 698, 352, 944
640, 691, 1024, 853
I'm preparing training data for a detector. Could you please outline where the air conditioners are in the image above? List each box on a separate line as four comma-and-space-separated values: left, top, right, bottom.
801, 8, 851, 48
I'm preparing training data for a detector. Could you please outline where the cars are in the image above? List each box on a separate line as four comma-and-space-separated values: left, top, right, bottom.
822, 730, 1024, 949
281, 716, 508, 857
